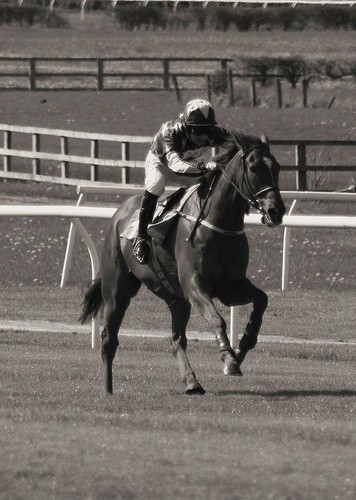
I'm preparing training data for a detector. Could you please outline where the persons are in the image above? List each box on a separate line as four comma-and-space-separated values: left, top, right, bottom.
134, 99, 269, 265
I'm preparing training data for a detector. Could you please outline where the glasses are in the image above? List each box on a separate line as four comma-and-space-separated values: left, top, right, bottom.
190, 129, 212, 136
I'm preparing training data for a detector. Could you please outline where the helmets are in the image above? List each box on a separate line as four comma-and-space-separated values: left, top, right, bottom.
184, 98, 217, 127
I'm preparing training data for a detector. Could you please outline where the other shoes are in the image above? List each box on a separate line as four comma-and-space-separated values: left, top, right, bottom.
134, 237, 148, 264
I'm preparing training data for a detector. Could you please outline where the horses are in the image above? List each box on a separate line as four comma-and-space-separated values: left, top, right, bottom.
73, 127, 289, 399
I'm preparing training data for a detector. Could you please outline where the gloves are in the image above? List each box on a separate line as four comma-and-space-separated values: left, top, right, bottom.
206, 161, 221, 172
